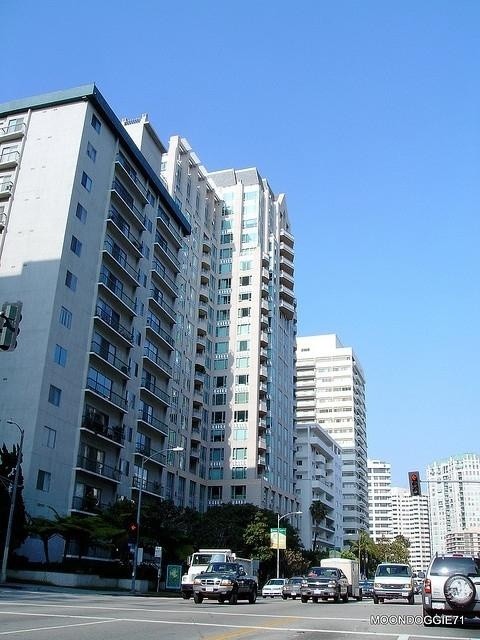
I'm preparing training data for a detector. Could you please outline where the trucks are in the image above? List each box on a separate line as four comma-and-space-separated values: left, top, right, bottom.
179, 545, 259, 600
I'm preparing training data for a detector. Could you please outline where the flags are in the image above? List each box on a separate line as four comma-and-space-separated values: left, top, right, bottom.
270, 527, 277, 549
279, 528, 287, 549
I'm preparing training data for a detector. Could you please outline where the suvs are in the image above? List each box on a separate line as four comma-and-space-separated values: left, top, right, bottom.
421, 551, 480, 623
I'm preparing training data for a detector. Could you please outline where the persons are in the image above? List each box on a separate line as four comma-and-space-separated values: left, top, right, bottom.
313, 571, 321, 577
380, 569, 391, 576
332, 573, 338, 577
238, 566, 248, 576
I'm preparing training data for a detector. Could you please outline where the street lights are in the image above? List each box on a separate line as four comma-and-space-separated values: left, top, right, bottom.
276, 510, 305, 579
0, 417, 26, 585
339, 533, 352, 553
127, 444, 187, 595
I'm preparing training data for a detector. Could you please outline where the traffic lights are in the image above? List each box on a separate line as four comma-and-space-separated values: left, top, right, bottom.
129, 521, 137, 544
408, 471, 421, 497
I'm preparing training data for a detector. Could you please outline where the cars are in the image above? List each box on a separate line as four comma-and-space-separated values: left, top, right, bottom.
261, 551, 424, 603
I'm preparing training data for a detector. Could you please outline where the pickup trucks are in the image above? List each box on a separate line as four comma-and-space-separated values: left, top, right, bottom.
192, 561, 258, 607
